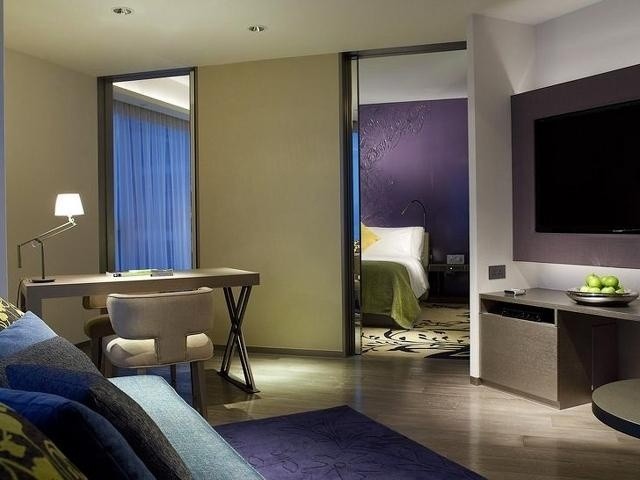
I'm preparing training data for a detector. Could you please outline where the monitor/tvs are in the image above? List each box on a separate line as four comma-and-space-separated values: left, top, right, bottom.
532, 98, 640, 235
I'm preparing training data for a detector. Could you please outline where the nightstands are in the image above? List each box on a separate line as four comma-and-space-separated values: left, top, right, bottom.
429, 264, 469, 309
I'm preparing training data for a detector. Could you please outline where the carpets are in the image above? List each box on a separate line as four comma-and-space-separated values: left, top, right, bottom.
213, 405, 490, 479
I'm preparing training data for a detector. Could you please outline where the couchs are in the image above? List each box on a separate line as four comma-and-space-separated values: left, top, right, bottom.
0, 296, 266, 479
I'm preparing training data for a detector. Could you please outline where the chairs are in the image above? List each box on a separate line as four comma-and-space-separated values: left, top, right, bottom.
82, 287, 215, 420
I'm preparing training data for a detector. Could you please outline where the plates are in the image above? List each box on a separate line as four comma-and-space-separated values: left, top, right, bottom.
566, 289, 638, 307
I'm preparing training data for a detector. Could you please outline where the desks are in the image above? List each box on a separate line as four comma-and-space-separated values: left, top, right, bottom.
19, 267, 261, 395
592, 379, 640, 439
480, 286, 640, 411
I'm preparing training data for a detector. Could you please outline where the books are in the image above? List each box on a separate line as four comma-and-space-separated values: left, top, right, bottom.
129, 268, 174, 273
105, 270, 174, 278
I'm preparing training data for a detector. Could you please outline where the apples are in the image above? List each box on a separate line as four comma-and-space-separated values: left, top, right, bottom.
580, 273, 626, 294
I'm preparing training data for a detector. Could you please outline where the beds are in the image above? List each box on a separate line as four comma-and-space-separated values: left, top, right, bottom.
361, 219, 429, 332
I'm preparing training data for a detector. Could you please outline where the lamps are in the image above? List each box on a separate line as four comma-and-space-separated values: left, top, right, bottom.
17, 188, 85, 283
401, 200, 427, 231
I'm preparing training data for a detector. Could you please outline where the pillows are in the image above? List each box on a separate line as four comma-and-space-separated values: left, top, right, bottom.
361, 222, 381, 253
0, 297, 194, 480
